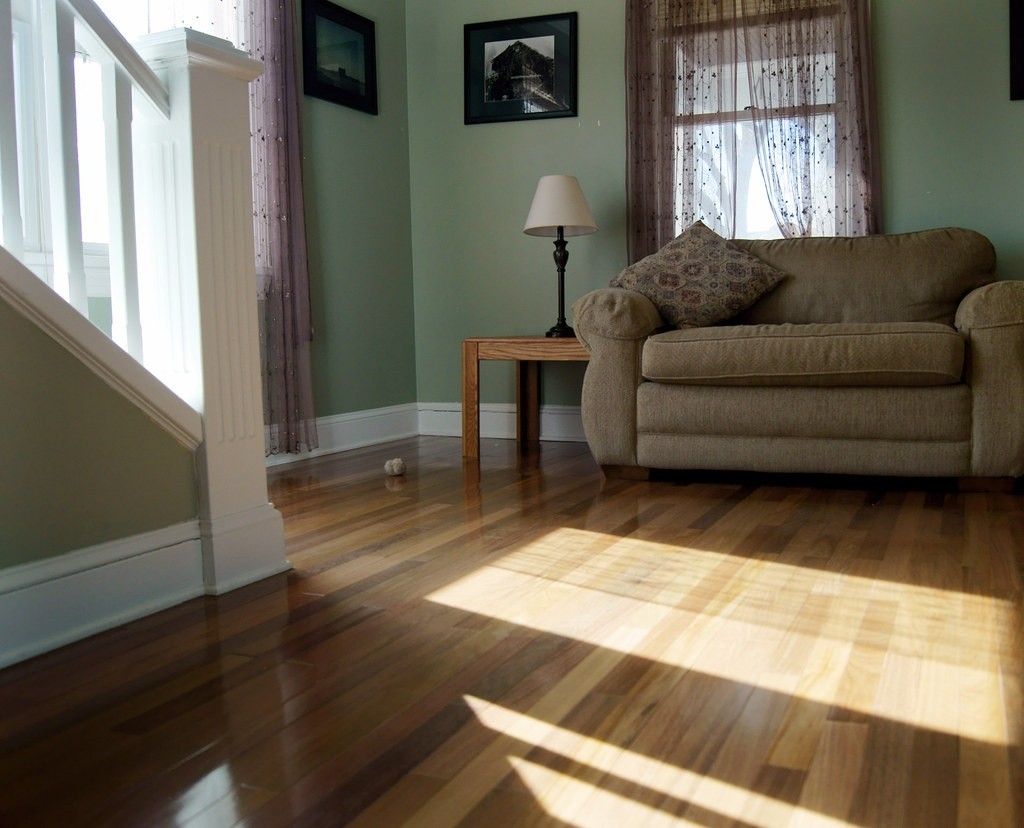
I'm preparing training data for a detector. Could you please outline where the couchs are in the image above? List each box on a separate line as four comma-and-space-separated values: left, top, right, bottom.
571, 228, 1023, 486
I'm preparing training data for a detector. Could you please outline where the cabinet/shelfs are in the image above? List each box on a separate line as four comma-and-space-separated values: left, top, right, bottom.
462, 334, 593, 469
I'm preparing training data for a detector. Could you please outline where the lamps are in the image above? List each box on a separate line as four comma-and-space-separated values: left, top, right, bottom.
522, 174, 596, 337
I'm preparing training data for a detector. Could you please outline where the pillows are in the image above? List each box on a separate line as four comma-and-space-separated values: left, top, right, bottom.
610, 218, 784, 330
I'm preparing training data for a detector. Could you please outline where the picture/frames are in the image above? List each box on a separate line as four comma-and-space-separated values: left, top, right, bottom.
464, 10, 580, 125
299, 0, 381, 115
